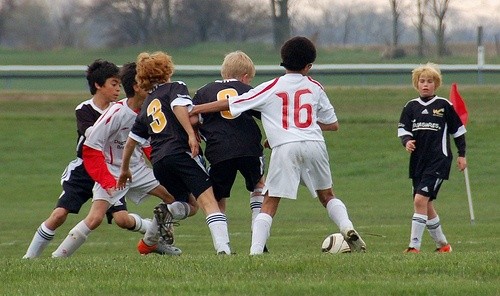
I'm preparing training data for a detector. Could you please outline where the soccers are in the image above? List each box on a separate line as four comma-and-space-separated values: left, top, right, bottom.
321, 232, 351, 254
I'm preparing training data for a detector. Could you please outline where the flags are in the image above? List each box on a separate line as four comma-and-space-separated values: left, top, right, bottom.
450, 84, 467, 125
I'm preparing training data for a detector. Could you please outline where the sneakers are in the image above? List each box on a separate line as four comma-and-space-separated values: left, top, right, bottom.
153, 203, 180, 245
435, 243, 452, 253
403, 247, 420, 253
138, 237, 182, 255
344, 226, 367, 254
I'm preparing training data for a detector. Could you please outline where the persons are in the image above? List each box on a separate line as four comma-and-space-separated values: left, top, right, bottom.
397, 63, 466, 252
51, 63, 182, 257
192, 51, 271, 252
116, 52, 230, 255
23, 58, 152, 258
189, 37, 366, 255
